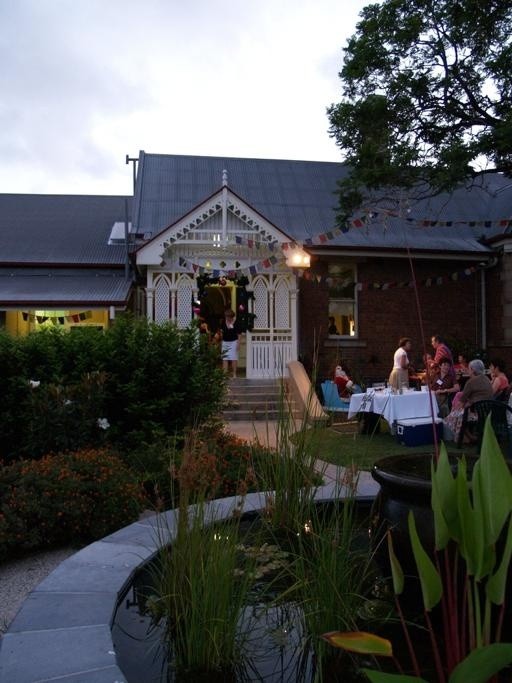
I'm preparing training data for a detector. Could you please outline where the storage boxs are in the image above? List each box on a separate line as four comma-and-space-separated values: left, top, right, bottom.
396, 417, 443, 447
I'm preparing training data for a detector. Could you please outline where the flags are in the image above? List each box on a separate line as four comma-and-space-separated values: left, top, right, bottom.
21, 309, 94, 326
289, 209, 380, 249
178, 233, 290, 279
383, 210, 511, 231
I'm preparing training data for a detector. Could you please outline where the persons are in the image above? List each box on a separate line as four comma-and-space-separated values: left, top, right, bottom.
199, 319, 211, 336
421, 351, 511, 443
329, 359, 354, 402
329, 315, 340, 335
387, 336, 415, 388
219, 308, 242, 378
425, 332, 454, 366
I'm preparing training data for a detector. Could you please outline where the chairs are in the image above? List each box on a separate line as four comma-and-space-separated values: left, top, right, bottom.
457, 385, 512, 455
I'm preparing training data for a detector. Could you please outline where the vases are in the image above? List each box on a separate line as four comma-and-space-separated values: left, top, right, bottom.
368, 453, 512, 577
